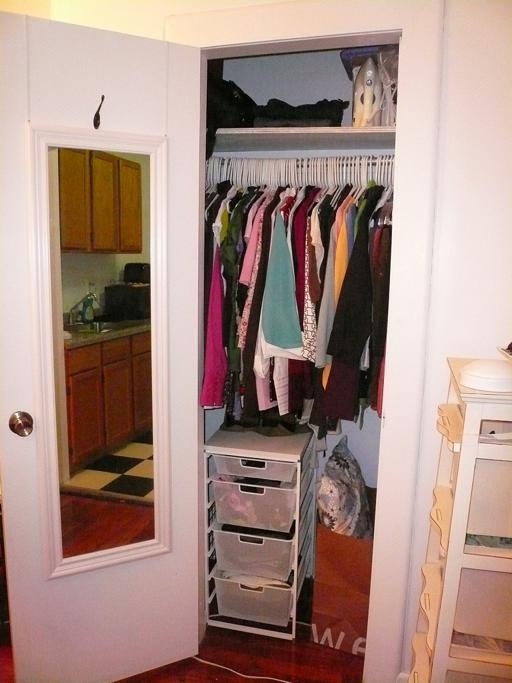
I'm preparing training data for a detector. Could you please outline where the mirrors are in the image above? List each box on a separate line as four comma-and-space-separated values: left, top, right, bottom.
33, 128, 173, 582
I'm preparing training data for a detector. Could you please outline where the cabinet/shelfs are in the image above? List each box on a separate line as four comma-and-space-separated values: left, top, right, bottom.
198, 30, 436, 683
403, 354, 511, 682
68, 332, 150, 464
56, 141, 143, 255
202, 415, 317, 641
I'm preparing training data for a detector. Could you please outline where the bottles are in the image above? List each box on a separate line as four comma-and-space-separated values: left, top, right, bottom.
84, 296, 93, 323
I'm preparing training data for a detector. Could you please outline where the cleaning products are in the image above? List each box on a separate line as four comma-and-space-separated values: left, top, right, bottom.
81, 293, 94, 323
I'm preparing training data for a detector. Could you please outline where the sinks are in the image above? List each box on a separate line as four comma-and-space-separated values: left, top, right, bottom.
64, 321, 115, 333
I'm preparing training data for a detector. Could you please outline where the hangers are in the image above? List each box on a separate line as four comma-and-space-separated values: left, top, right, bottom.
200, 148, 397, 229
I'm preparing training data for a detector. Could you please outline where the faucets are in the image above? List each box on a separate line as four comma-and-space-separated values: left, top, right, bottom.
67, 291, 97, 324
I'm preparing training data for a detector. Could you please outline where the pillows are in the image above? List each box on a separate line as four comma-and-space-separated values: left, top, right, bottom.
314, 436, 370, 539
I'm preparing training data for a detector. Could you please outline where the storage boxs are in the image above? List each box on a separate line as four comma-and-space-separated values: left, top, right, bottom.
310, 484, 377, 657
